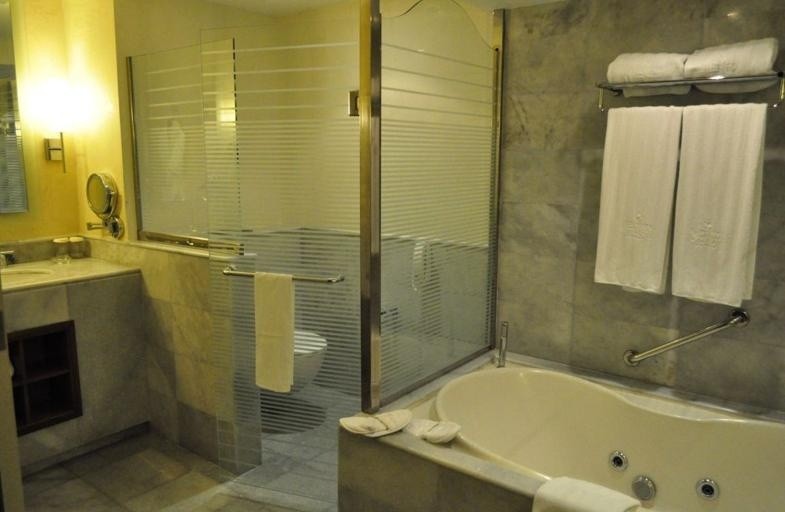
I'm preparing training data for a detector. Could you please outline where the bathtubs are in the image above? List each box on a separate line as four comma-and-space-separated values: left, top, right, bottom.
382, 328, 432, 400
437, 364, 784, 512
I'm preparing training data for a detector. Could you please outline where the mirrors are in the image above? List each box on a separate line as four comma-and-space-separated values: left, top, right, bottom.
0, 27, 30, 215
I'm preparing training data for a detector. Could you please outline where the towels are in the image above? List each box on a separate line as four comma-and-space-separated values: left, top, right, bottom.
594, 105, 684, 300
668, 102, 768, 308
251, 271, 295, 392
685, 37, 783, 95
531, 475, 641, 512
606, 50, 692, 98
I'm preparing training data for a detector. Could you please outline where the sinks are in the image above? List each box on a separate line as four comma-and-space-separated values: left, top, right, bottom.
0, 268, 54, 286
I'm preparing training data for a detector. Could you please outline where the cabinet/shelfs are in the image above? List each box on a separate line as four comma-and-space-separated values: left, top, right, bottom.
1, 273, 149, 478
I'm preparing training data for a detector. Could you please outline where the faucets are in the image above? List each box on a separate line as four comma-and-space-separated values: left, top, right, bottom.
497, 320, 510, 367
1, 250, 15, 265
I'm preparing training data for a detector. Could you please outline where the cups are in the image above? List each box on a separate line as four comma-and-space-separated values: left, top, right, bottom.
52, 237, 87, 260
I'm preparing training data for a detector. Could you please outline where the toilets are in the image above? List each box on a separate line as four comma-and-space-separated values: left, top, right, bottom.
262, 327, 329, 396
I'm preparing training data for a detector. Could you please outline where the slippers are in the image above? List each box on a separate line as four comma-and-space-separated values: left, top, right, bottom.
340, 409, 411, 438
402, 419, 461, 444
